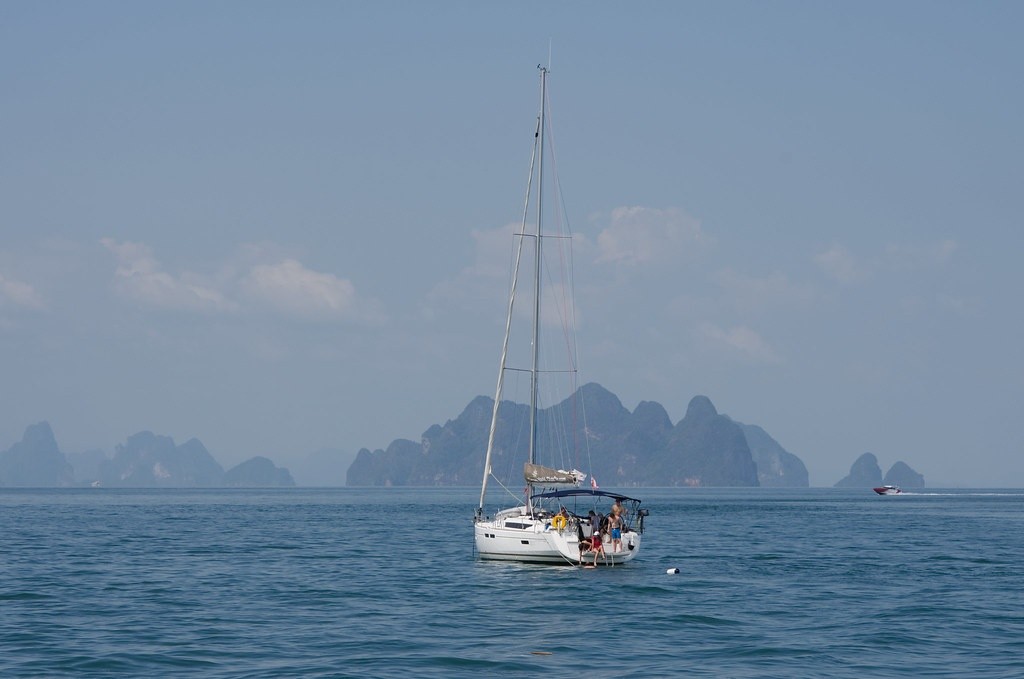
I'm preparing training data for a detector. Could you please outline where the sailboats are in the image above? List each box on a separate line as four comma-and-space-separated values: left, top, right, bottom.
472, 40, 649, 565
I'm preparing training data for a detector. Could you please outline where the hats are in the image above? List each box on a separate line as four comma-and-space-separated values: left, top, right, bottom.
594, 531, 600, 536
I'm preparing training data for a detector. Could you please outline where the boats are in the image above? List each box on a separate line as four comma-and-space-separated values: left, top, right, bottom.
873, 484, 903, 495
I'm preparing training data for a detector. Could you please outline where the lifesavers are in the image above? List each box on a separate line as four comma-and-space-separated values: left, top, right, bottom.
551, 515, 566, 529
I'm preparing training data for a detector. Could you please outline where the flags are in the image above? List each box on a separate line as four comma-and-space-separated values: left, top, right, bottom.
591, 474, 600, 490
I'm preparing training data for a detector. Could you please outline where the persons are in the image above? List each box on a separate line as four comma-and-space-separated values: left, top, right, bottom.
556, 499, 627, 567
